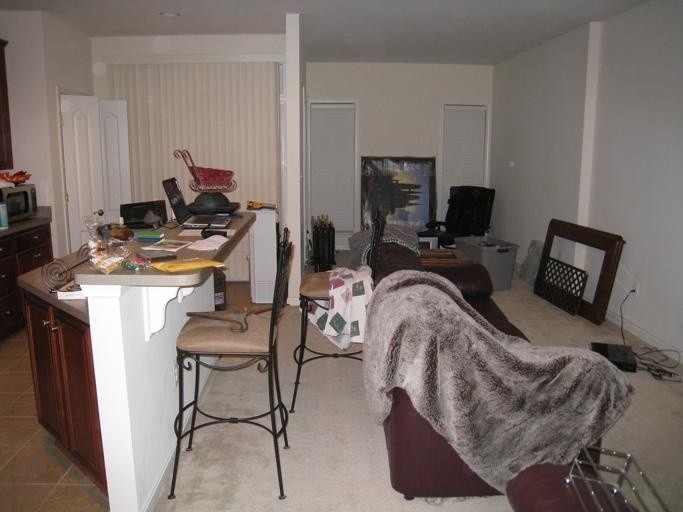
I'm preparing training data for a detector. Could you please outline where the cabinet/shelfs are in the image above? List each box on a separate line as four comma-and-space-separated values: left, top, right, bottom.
0, 212, 256, 512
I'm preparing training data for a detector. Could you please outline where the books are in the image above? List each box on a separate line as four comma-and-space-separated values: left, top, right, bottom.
56, 280, 86, 300
135, 249, 177, 263
135, 230, 164, 238
141, 239, 190, 252
148, 257, 225, 275
134, 236, 163, 243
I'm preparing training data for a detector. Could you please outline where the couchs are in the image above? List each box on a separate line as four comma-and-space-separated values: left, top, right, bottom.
347, 227, 639, 512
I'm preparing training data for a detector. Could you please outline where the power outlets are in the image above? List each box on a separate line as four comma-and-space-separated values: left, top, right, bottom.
630, 282, 641, 296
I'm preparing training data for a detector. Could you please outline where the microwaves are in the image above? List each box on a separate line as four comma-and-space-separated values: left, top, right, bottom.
0, 183, 38, 224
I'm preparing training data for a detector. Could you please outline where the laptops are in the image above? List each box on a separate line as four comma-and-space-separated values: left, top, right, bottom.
162, 177, 232, 229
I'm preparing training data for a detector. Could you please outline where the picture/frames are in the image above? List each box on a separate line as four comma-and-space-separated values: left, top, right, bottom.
360, 155, 437, 233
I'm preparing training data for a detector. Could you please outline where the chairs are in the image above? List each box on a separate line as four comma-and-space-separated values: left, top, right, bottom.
166, 227, 293, 500
418, 185, 495, 246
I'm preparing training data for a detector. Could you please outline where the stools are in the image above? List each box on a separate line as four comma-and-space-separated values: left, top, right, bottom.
289, 270, 372, 412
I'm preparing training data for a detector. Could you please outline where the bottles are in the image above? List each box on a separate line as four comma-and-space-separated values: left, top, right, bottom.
0, 201, 9, 230
